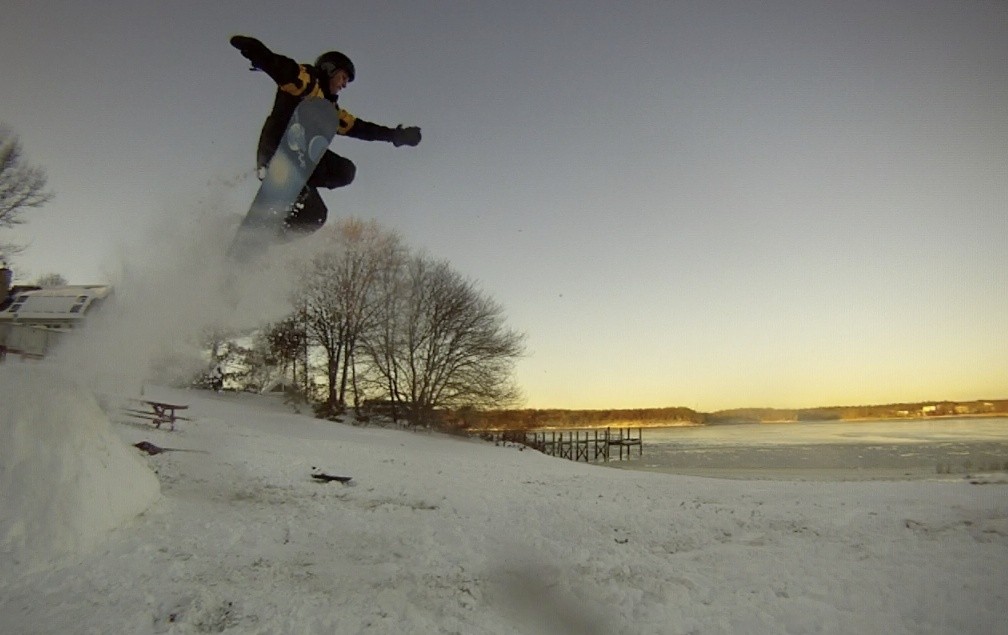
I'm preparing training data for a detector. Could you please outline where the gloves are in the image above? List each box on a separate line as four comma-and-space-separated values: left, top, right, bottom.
393, 123, 422, 147
231, 35, 266, 60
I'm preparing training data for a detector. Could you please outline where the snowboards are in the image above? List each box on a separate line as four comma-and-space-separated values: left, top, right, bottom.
228, 94, 340, 255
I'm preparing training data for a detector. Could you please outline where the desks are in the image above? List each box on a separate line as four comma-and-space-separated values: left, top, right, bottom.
116, 397, 189, 433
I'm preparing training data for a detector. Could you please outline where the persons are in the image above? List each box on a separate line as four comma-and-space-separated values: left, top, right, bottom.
231, 35, 423, 249
0, 268, 15, 362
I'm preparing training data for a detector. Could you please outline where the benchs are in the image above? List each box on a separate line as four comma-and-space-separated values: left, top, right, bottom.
124, 408, 191, 430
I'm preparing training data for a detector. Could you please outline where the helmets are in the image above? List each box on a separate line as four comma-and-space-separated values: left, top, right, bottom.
315, 50, 355, 81
